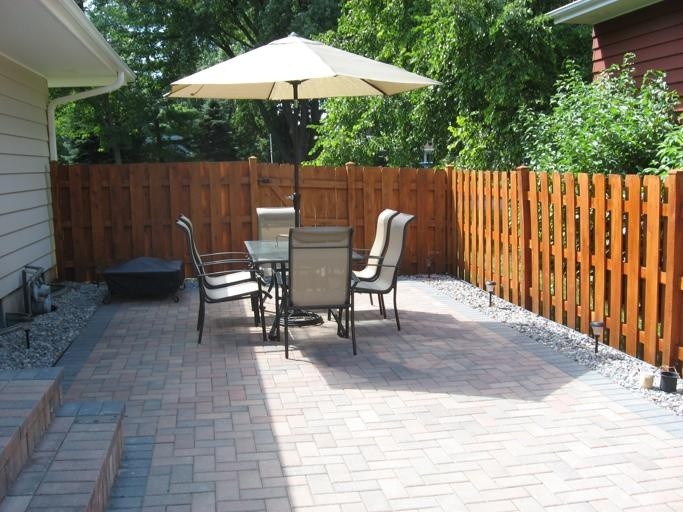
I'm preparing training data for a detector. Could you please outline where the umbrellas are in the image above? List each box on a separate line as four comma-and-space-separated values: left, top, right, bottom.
161, 31, 443, 227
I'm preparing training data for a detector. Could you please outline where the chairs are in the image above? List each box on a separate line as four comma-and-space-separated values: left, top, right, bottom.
175, 206, 417, 358
